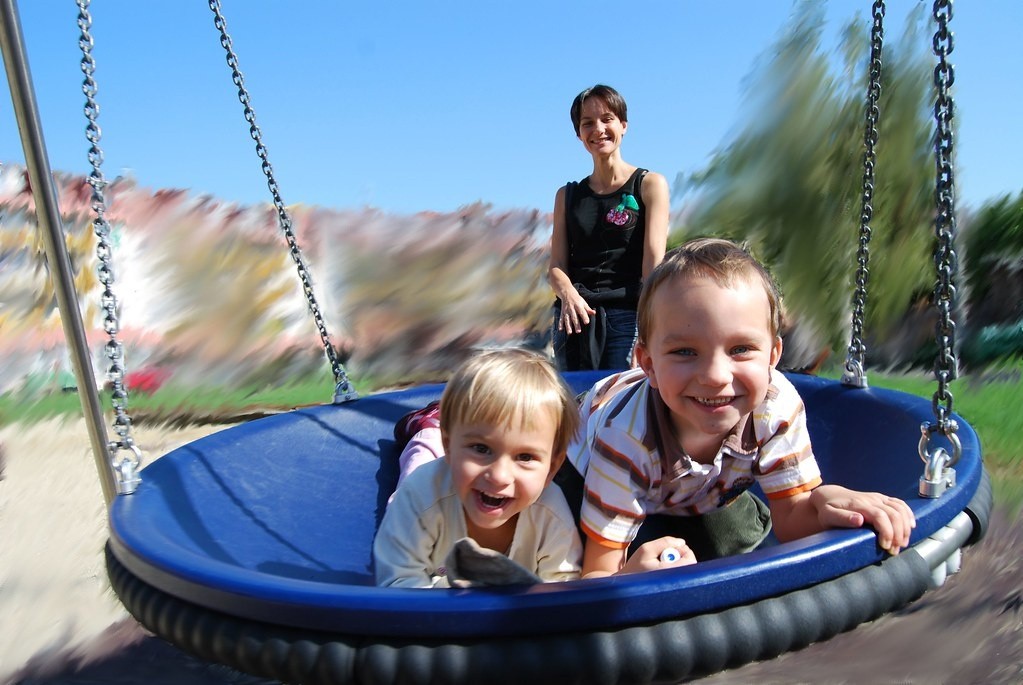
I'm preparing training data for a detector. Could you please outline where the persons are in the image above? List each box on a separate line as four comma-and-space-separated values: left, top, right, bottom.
561, 236, 917, 584
372, 344, 582, 592
547, 84, 671, 371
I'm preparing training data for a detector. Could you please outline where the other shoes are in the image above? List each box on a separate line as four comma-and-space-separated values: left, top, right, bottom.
394, 400, 440, 442
